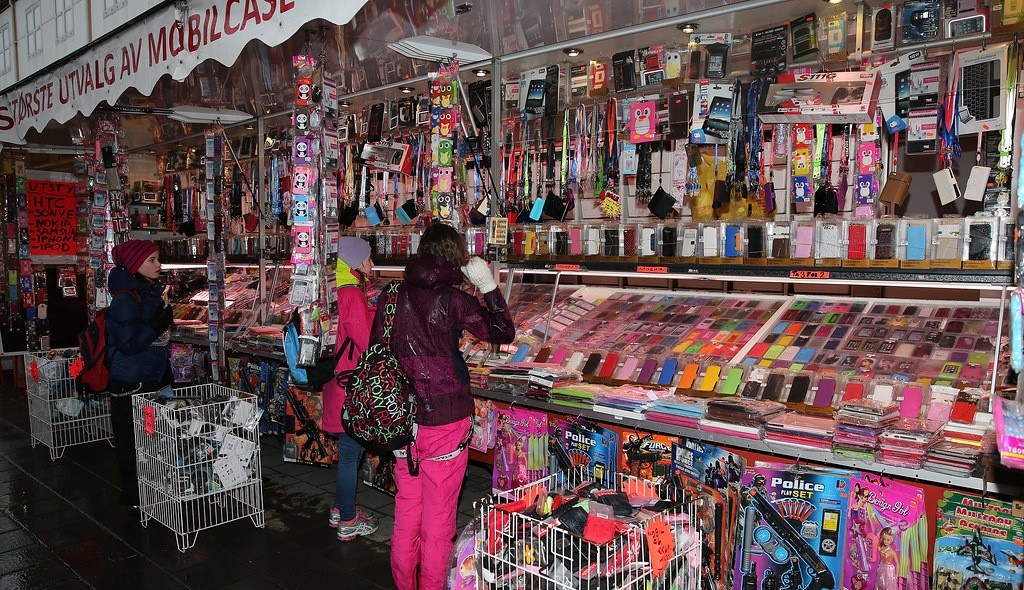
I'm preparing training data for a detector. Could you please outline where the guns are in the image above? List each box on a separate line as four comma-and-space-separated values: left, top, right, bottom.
779, 558, 804, 590
285, 389, 329, 461
749, 487, 835, 590
551, 437, 581, 486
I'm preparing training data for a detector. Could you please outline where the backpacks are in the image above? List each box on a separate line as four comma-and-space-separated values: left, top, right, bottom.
280, 306, 354, 394
75, 289, 141, 393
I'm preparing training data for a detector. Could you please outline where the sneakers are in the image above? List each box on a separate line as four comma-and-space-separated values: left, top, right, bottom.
328, 505, 373, 528
337, 512, 380, 541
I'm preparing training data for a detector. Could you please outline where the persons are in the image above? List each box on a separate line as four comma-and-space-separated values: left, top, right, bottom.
319, 236, 379, 541
103, 240, 174, 503
368, 223, 515, 590
874, 528, 899, 590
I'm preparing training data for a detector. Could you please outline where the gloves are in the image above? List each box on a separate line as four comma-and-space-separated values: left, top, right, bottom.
460, 255, 498, 295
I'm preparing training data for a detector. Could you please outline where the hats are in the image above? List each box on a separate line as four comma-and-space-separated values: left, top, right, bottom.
111, 239, 158, 275
337, 236, 371, 269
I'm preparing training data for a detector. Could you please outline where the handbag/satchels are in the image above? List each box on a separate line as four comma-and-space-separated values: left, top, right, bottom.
341, 279, 417, 454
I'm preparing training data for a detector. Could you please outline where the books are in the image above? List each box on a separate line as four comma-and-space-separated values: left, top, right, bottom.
469, 362, 1024, 478
